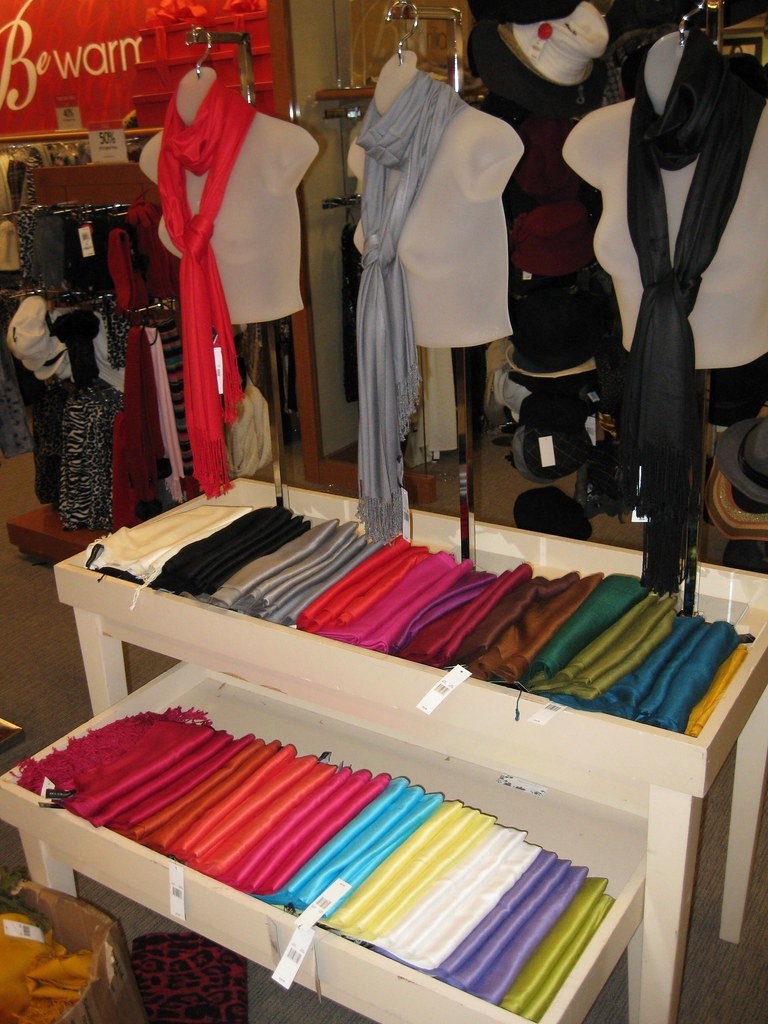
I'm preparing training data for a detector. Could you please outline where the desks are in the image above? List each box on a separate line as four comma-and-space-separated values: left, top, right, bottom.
1, 662, 647, 1024
53, 471, 768, 1024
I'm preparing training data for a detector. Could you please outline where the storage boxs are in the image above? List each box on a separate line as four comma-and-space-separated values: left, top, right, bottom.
8, 877, 153, 1024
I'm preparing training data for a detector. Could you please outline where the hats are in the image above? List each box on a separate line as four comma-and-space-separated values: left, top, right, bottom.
466, 0, 768, 576
6, 294, 69, 379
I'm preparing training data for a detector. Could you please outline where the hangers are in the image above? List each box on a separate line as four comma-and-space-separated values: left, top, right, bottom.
0, 137, 144, 158
23, 287, 178, 324
52, 203, 124, 231
